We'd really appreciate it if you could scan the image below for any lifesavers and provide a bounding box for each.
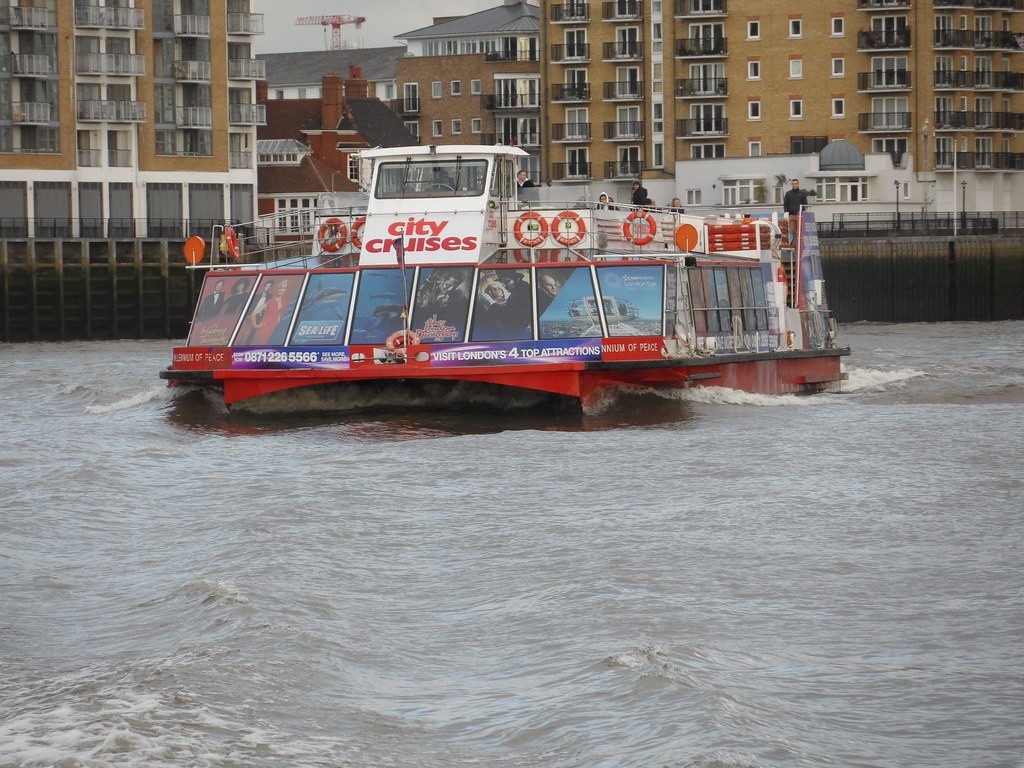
[514,250,548,263]
[226,227,240,259]
[386,330,420,354]
[350,217,366,249]
[317,218,347,253]
[551,249,584,262]
[623,212,656,245]
[514,212,548,247]
[551,211,585,245]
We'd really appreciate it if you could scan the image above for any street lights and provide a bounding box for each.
[894,180,901,212]
[961,180,967,211]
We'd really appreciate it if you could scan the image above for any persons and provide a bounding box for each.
[669,200,683,214]
[596,192,610,210]
[572,196,588,211]
[431,268,564,311]
[607,197,617,211]
[631,180,648,209]
[513,170,535,188]
[434,172,457,191]
[195,277,290,347]
[784,180,809,217]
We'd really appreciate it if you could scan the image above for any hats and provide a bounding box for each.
[631,178,642,189]
[493,269,524,281]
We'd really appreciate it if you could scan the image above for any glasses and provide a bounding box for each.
[500,279,512,284]
[279,287,287,289]
[442,176,448,178]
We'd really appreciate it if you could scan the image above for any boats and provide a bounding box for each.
[157,137,850,419]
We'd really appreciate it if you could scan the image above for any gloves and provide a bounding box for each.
[783,212,790,223]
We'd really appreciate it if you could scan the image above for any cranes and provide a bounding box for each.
[294,15,365,51]
